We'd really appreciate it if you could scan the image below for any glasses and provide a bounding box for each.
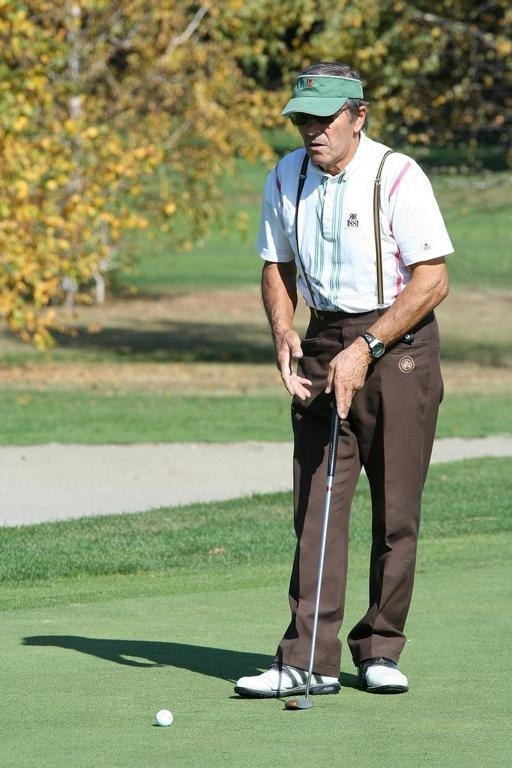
[286,106,358,128]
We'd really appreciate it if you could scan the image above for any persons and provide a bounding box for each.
[231,62,455,702]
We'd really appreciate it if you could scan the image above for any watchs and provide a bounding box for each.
[360,330,387,361]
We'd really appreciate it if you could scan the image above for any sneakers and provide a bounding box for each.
[232,659,344,699]
[358,655,411,694]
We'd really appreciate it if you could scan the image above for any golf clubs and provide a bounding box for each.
[285,389,340,710]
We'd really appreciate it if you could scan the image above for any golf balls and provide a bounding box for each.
[156,709,173,726]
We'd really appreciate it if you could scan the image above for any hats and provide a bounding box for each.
[281,72,365,119]
